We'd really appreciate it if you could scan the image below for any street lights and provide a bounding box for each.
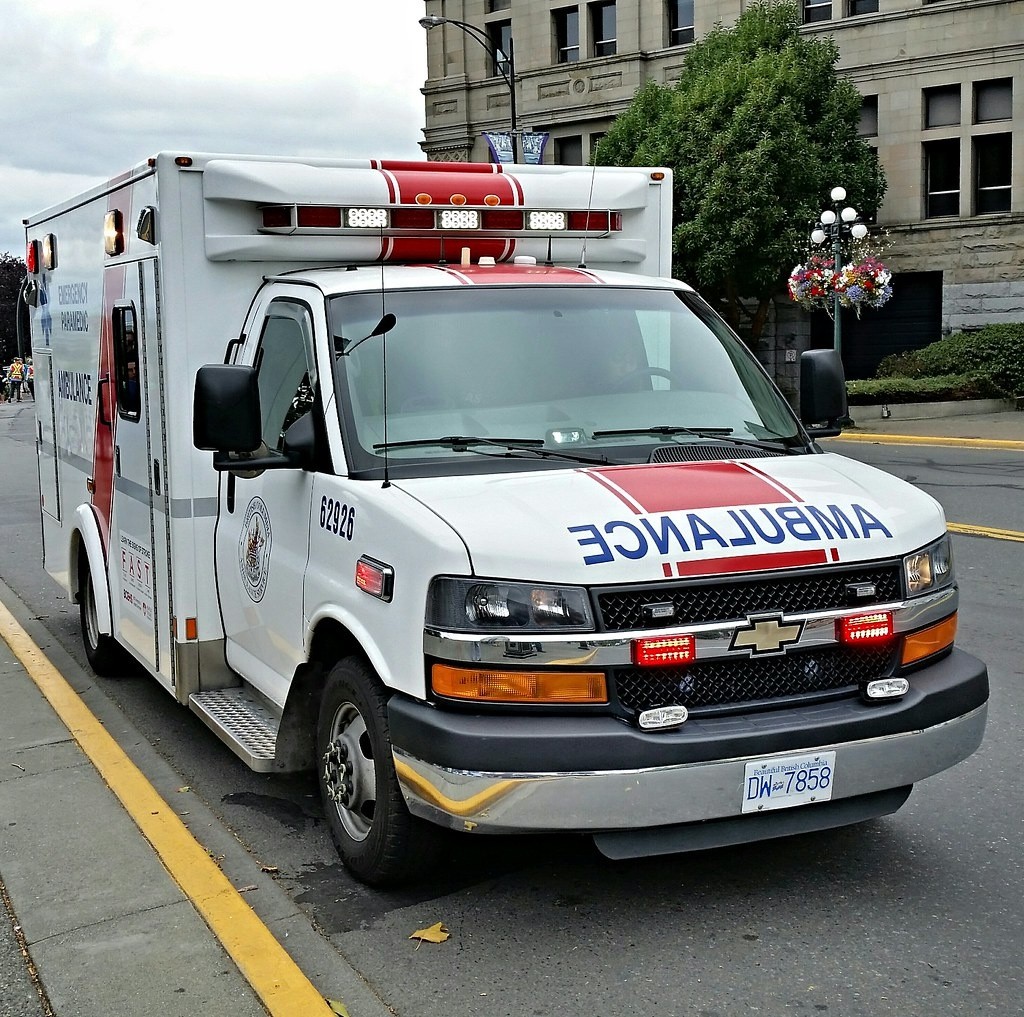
[418,17,517,164]
[811,187,867,359]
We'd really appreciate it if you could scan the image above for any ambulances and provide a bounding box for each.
[21,152,987,891]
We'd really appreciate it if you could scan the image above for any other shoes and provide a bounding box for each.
[17,400,22,402]
[22,391,29,394]
[8,397,11,403]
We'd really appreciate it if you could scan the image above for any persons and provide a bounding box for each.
[0,356,35,402]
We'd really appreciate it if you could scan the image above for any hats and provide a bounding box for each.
[14,357,20,361]
[28,359,33,364]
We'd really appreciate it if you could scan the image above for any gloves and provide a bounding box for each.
[21,377,24,381]
[8,377,11,380]
